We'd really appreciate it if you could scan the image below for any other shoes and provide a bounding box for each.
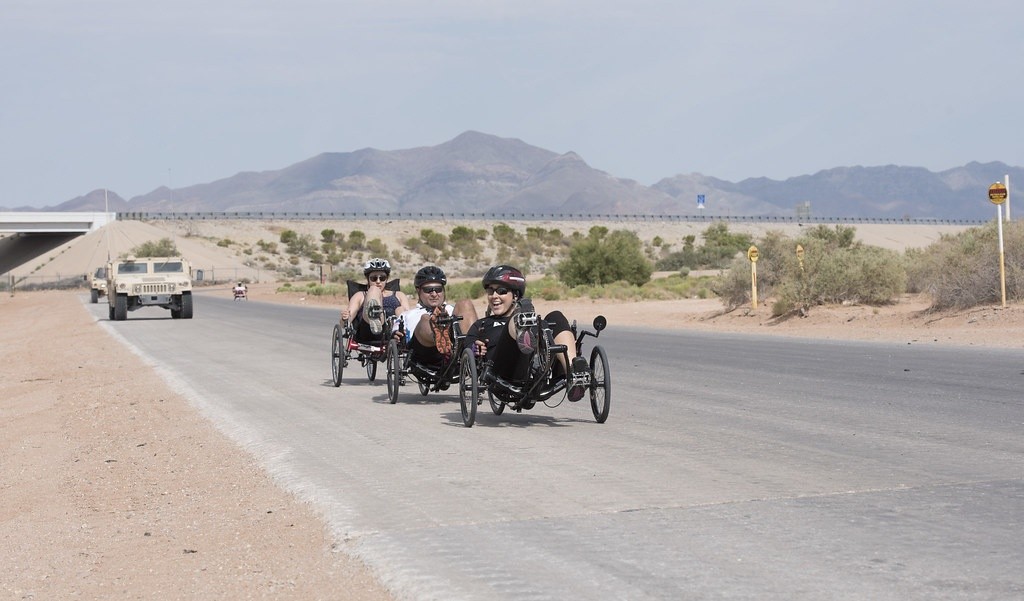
[366,298,383,335]
[429,305,451,354]
[567,357,589,402]
[514,298,538,355]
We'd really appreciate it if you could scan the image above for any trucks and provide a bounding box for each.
[104,254,194,320]
[89,266,108,304]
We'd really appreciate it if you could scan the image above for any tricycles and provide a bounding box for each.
[379,305,508,416]
[233,285,248,301]
[458,313,612,428]
[330,277,411,388]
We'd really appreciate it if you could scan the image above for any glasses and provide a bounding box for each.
[485,286,512,295]
[420,286,445,292]
[367,275,389,281]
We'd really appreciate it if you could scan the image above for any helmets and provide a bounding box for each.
[482,265,525,297]
[364,258,390,276]
[414,266,447,289]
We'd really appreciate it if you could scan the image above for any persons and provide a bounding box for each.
[234,282,245,290]
[339,258,410,350]
[391,266,480,367]
[463,265,590,402]
